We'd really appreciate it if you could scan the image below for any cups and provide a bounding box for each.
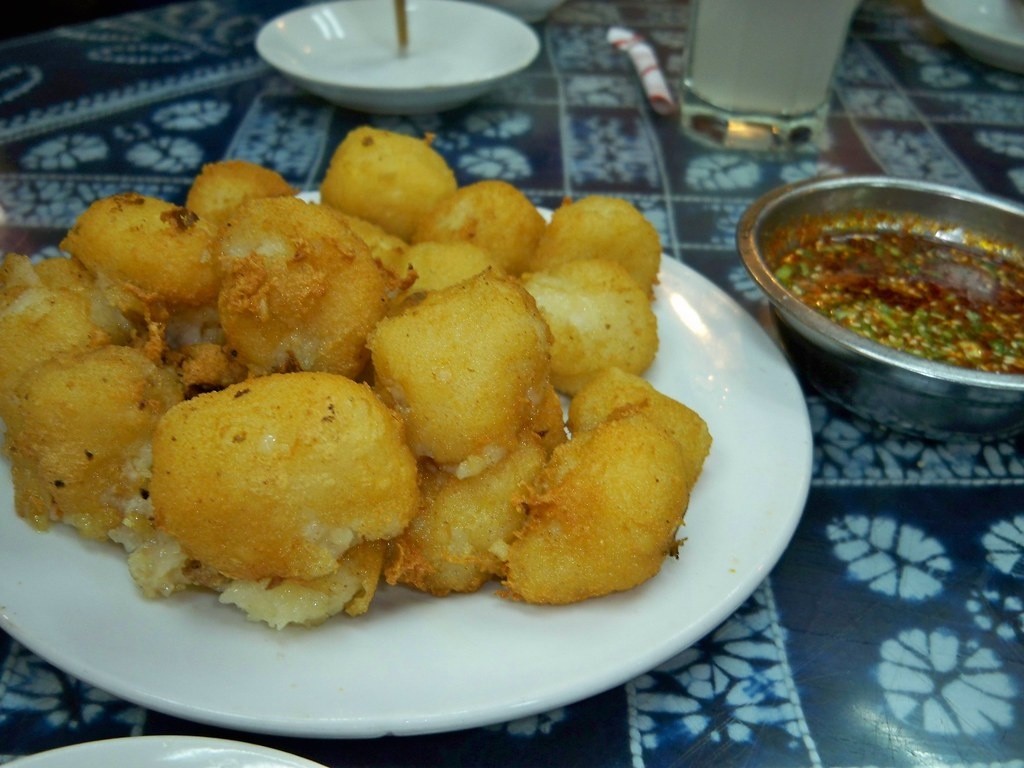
[679,0,861,153]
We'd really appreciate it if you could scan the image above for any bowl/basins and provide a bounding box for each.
[736,172,1024,442]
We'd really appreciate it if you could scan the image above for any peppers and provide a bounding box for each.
[773,237,1024,377]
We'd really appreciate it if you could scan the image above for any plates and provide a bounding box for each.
[0,197,816,741]
[921,0,1024,75]
[0,735,326,768]
[253,0,541,116]
